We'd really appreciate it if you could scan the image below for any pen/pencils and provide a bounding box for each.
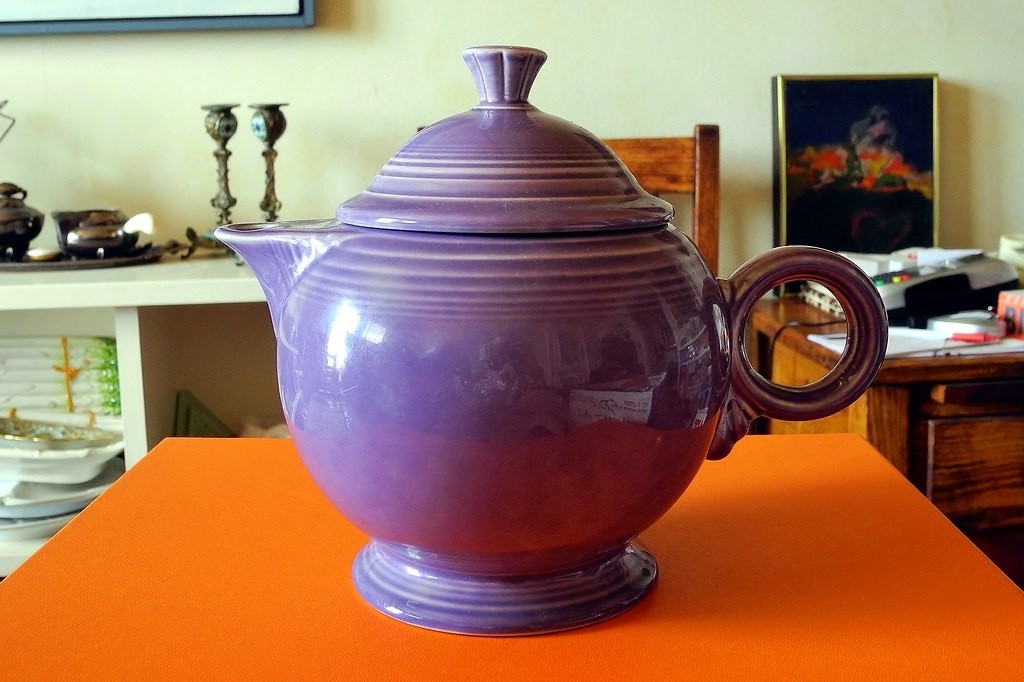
[887,328,998,342]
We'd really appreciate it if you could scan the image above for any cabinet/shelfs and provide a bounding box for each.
[0,251,270,582]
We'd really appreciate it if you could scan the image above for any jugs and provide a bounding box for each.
[213,44,888,636]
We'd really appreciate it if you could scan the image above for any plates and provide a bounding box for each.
[0,515,81,540]
[0,457,126,519]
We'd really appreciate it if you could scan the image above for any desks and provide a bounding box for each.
[744,297,1022,537]
[0,433,1024,682]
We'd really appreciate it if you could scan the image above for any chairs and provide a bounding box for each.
[416,125,720,279]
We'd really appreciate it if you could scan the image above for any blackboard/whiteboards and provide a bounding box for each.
[0,0,319,33]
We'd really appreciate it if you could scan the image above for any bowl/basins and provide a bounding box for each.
[0,409,124,484]
